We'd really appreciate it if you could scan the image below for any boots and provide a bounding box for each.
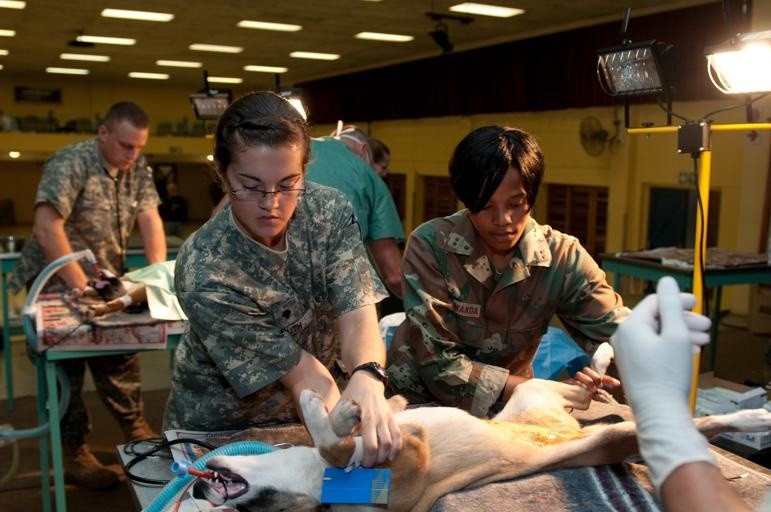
[59,436,121,488]
[125,419,160,439]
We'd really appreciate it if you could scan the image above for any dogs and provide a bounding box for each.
[66,269,148,319]
[191,340,771,512]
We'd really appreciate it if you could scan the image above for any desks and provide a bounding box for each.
[601,247,769,367]
[19,305,187,512]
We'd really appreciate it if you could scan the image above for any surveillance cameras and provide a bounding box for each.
[426,31,453,53]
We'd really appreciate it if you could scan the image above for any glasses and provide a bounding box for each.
[218,160,310,204]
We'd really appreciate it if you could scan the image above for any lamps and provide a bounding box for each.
[705,27,771,141]
[586,41,684,124]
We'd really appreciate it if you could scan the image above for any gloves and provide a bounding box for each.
[608,275,721,499]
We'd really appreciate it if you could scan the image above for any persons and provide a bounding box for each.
[19,100,166,488]
[391,124,647,420]
[604,261,749,511]
[161,88,399,464]
[369,139,393,176]
[305,123,410,301]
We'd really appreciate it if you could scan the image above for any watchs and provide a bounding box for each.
[352,359,389,384]
